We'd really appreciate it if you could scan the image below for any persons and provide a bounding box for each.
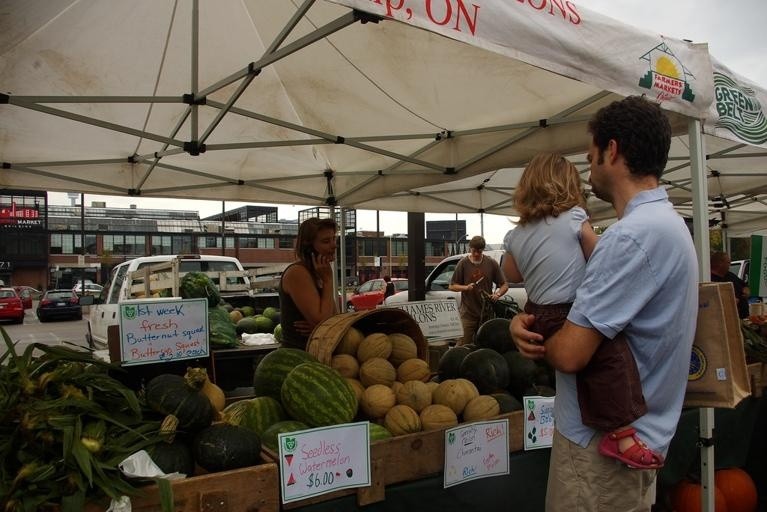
[499,152,665,469]
[383,275,395,298]
[709,251,750,320]
[508,94,699,512]
[280,217,339,347]
[447,236,510,346]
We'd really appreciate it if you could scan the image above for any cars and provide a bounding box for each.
[720,250,753,283]
[1,274,103,324]
[335,274,410,313]
[386,249,530,312]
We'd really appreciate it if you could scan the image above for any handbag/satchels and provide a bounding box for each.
[682,282,752,409]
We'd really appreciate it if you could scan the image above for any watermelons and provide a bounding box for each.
[209,308,237,347]
[264,306,277,321]
[331,324,501,437]
[241,305,254,317]
[181,272,220,307]
[236,317,257,336]
[218,298,233,311]
[223,348,392,455]
[257,315,274,331]
[274,324,284,343]
[437,317,555,413]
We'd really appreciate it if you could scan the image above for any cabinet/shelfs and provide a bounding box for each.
[40,324,767,512]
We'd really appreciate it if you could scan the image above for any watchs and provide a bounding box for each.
[496,292,500,297]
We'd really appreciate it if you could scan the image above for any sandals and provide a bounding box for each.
[598,427,665,469]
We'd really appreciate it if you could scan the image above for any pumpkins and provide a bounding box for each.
[714,464,758,512]
[126,367,260,486]
[670,479,727,512]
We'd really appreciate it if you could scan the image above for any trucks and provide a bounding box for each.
[86,253,257,354]
